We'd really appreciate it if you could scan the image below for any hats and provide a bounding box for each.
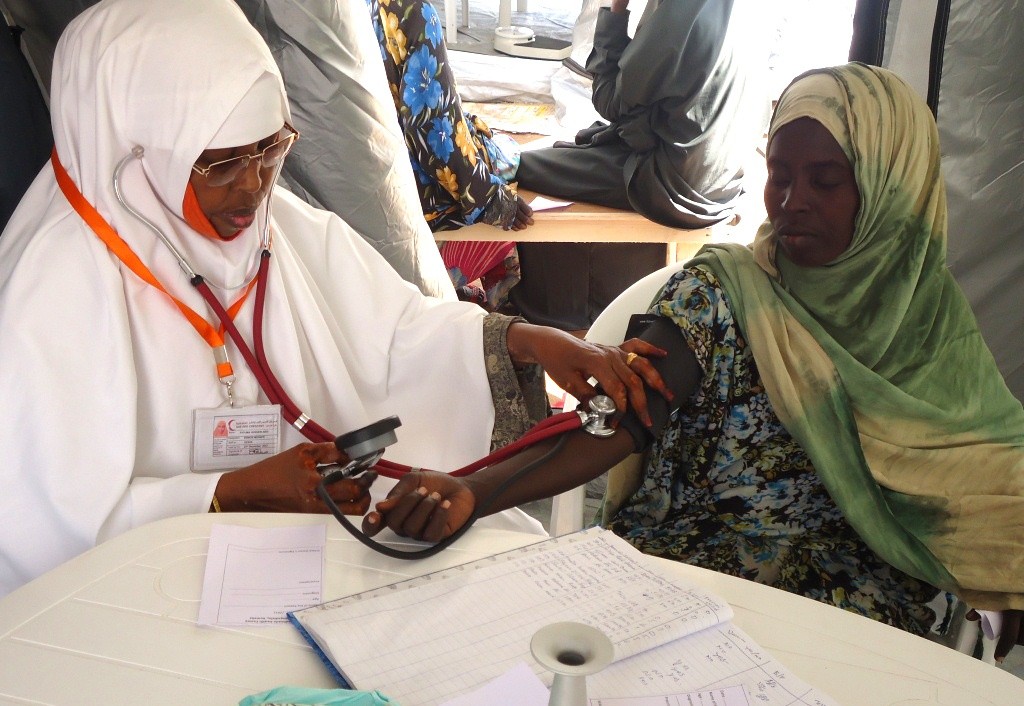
[200,72,285,150]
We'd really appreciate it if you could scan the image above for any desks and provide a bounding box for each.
[420,96,708,269]
[0,512,1024,706]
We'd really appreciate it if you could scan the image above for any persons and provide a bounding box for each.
[364,0,534,231]
[515,0,746,230]
[0,0,674,599]
[362,61,1024,662]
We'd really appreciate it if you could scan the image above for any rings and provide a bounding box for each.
[627,352,637,365]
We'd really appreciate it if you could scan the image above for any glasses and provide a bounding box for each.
[191,123,300,187]
